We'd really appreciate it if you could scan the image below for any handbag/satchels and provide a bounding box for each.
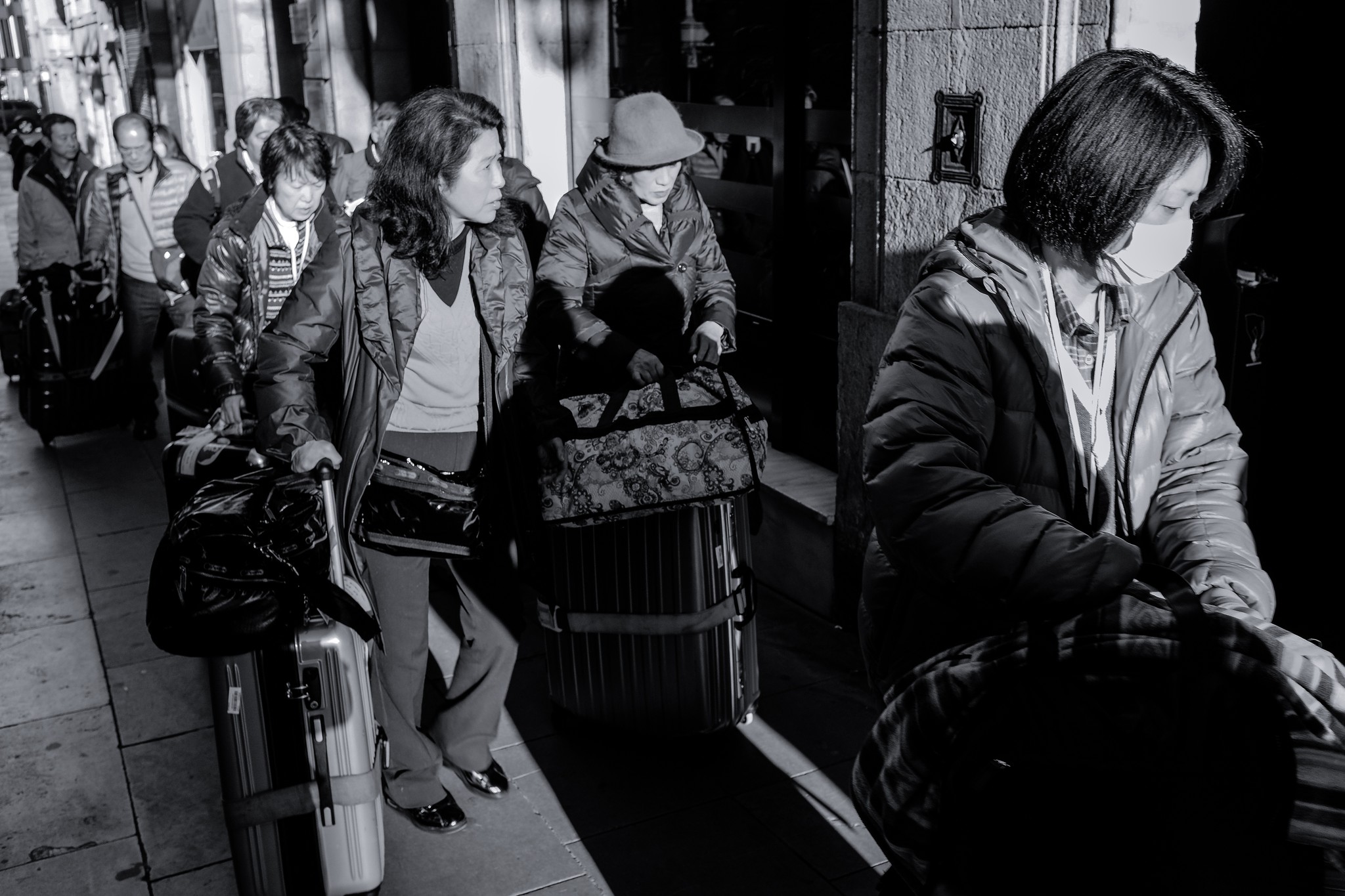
[146,465,331,660]
[526,355,770,532]
[847,563,1302,889]
[351,395,518,562]
[125,169,190,294]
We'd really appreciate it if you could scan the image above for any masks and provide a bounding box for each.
[1094,219,1194,288]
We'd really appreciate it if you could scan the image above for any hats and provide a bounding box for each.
[594,92,706,166]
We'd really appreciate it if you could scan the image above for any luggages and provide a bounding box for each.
[160,406,269,523]
[513,495,761,737]
[211,467,390,896]
[0,258,161,449]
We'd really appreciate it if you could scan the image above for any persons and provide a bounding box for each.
[10,94,554,427]
[531,89,741,390]
[850,47,1277,722]
[239,88,536,837]
